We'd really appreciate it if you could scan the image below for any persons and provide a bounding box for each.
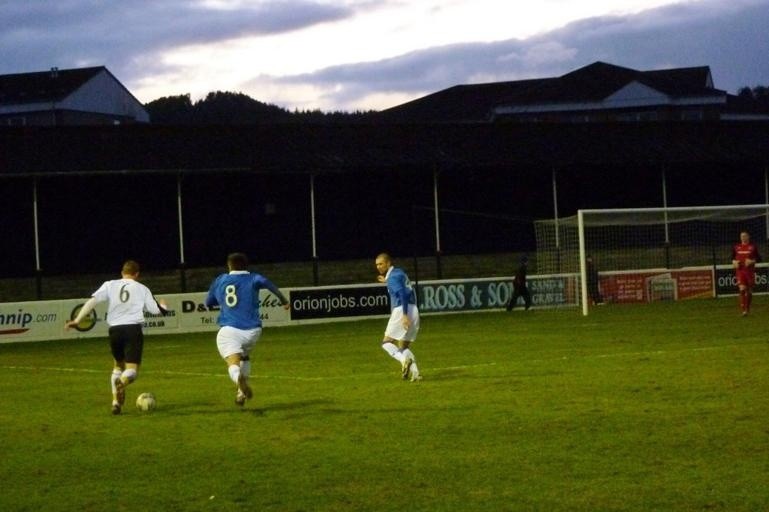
[506,256,535,312]
[731,231,762,317]
[64,261,167,415]
[205,253,290,406]
[373,252,424,382]
[586,254,605,306]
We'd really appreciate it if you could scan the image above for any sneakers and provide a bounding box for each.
[236,373,252,405]
[401,357,423,382]
[111,377,125,414]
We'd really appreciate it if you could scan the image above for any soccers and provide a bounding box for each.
[135,393,156,414]
